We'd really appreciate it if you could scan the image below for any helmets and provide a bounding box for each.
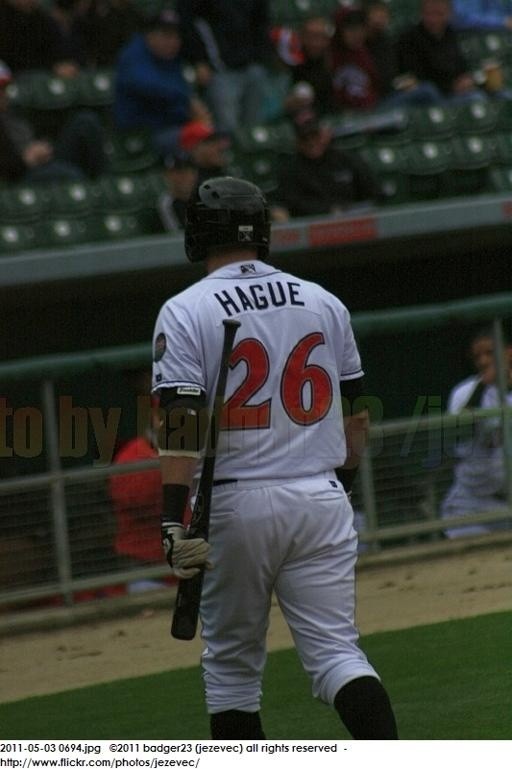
[183,175,273,264]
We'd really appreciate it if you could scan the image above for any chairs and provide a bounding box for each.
[0,0,512,257]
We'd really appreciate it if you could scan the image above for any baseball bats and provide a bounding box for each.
[170,320,243,640]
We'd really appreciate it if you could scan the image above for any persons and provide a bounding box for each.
[1,0,509,236]
[106,388,176,592]
[150,171,399,740]
[438,329,512,543]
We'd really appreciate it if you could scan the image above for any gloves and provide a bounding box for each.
[160,520,216,579]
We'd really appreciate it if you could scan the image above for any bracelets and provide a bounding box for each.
[159,480,191,525]
